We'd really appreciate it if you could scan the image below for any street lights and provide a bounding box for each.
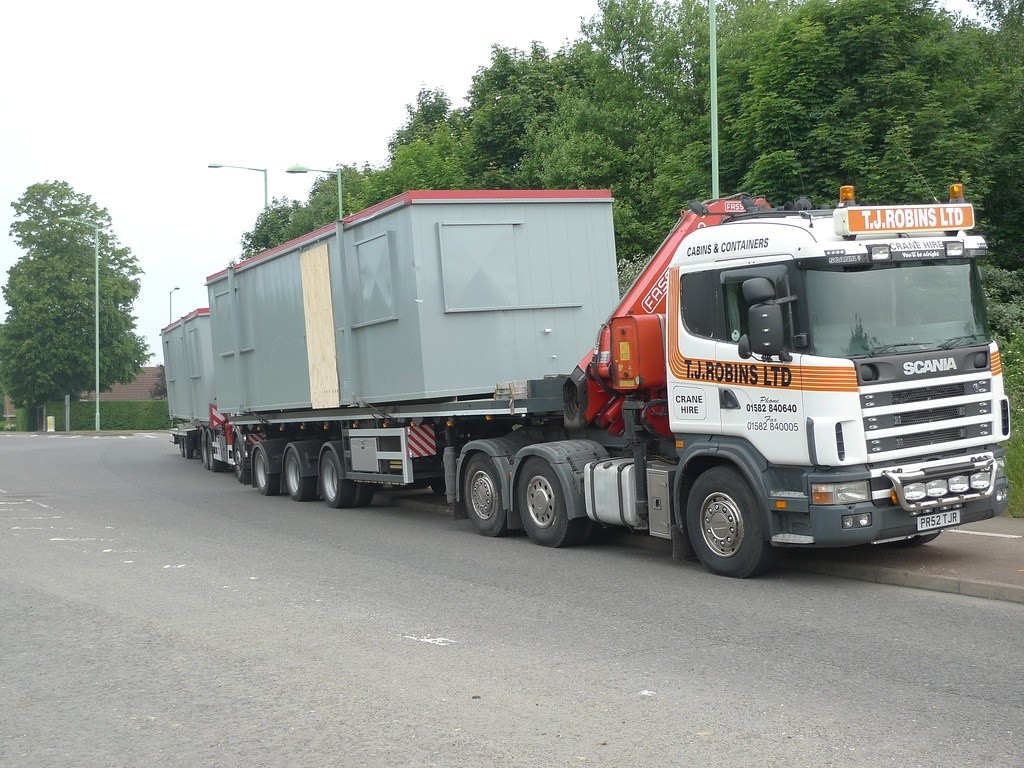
[210,163,268,215]
[285,167,344,218]
[170,287,178,323]
[58,218,100,434]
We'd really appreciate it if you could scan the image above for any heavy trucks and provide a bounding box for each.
[160,188,1011,582]
